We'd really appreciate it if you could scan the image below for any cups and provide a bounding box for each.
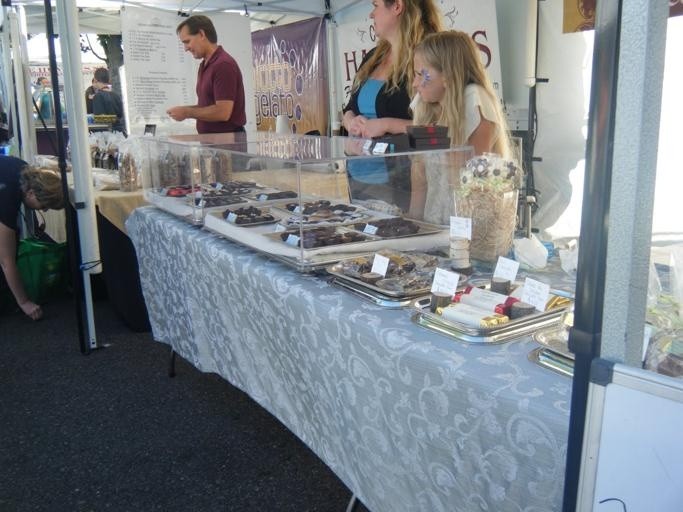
[276,115,289,135]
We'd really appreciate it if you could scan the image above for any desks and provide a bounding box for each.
[124,204,575,510]
[33,160,350,237]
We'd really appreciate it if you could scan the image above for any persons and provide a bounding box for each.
[31,76,56,121]
[340,0,444,217]
[165,15,248,182]
[406,29,513,228]
[0,154,64,321]
[92,68,127,137]
[85,78,95,114]
